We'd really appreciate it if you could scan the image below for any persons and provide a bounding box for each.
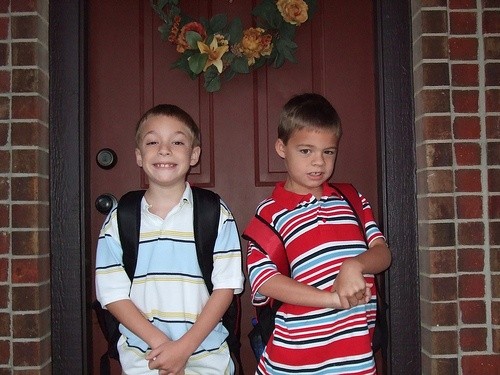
[96,103,245,375]
[239,91,393,375]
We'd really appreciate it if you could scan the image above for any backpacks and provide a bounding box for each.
[90,184,242,375]
[241,181,370,368]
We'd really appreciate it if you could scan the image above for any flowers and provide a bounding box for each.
[159,1,316,91]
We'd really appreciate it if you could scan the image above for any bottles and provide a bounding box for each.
[251,318,264,362]
